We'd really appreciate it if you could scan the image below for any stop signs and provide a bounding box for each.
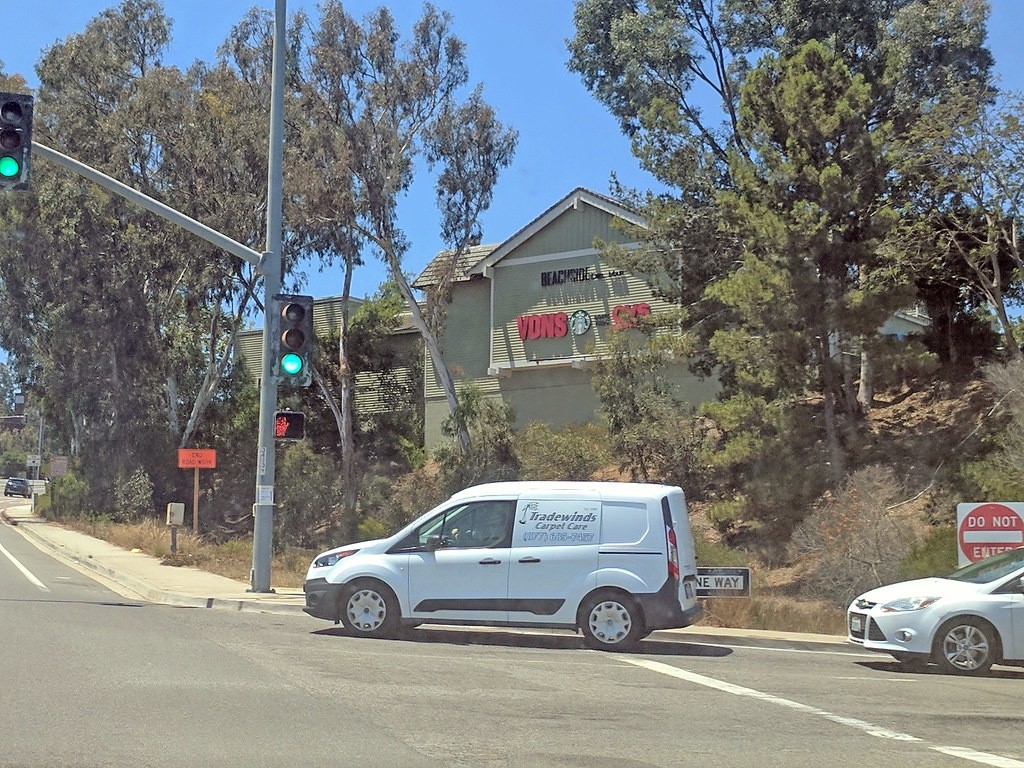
[956,502,1024,569]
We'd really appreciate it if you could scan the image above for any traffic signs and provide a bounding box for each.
[695,565,751,599]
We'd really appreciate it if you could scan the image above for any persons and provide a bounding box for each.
[452,512,507,546]
[45,477,51,486]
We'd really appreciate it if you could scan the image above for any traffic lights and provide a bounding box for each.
[276,292,313,388]
[0,93,35,190]
[272,411,306,444]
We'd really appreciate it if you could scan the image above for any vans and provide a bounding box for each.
[302,481,705,653]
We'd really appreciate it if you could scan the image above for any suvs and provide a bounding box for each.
[4,478,33,498]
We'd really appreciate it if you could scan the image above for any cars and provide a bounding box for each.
[846,547,1024,677]
